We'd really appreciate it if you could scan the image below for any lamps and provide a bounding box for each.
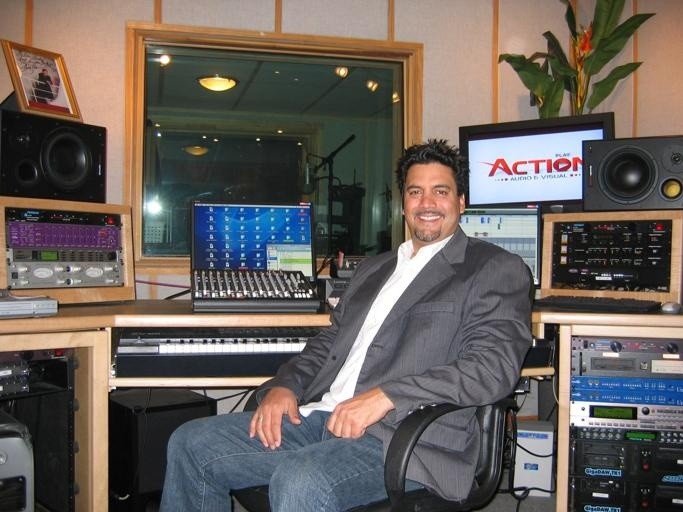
[184,145,208,156]
[195,75,238,92]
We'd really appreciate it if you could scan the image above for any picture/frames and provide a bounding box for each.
[0,39,83,123]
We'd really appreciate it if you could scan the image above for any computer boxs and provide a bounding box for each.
[0,409,37,512]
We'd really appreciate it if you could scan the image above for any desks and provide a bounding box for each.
[0,298,682,512]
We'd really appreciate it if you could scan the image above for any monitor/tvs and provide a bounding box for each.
[459,111,615,214]
[458,208,541,294]
[189,198,317,287]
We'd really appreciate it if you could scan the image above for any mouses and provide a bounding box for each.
[663,300,682,314]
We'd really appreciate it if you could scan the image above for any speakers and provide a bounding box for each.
[1,107,108,204]
[581,134,683,212]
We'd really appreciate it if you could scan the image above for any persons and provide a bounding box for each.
[160,138,534,511]
[35,68,54,103]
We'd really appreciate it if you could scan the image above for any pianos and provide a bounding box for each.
[115,326,321,378]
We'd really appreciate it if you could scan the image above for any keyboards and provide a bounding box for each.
[532,294,661,314]
